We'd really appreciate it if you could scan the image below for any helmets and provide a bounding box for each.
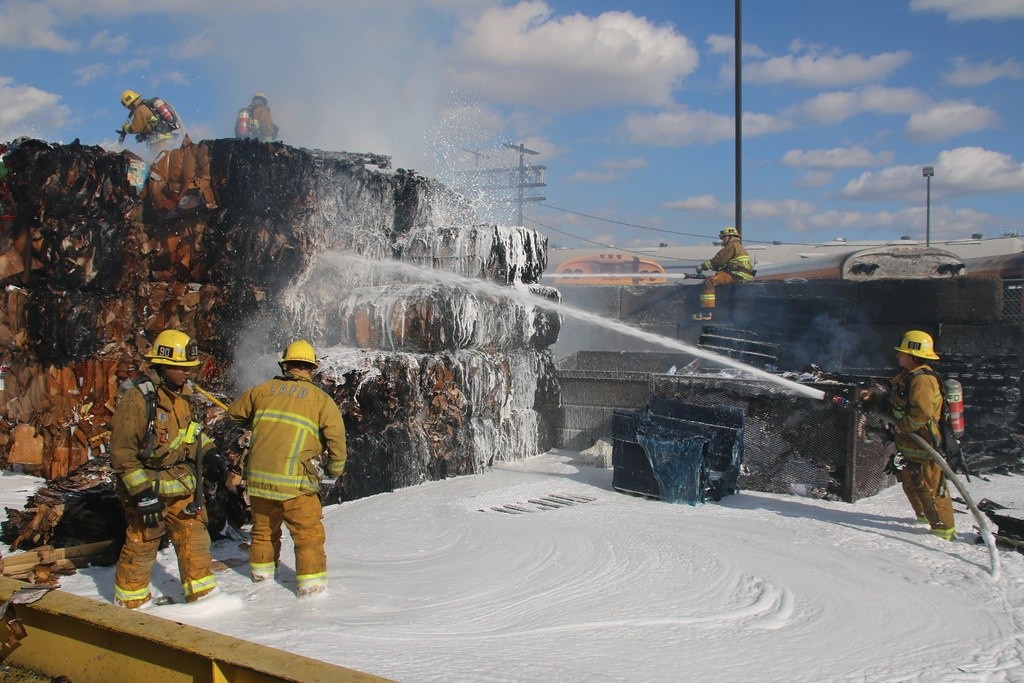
[894,329,941,360]
[719,227,741,240]
[120,91,141,110]
[252,92,268,106]
[278,339,319,369]
[145,329,200,367]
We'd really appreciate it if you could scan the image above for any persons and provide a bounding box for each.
[118,90,178,159]
[108,329,220,612]
[235,93,277,143]
[225,339,347,595]
[691,226,757,321]
[860,329,961,542]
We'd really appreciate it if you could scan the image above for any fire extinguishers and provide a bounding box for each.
[944,378,966,444]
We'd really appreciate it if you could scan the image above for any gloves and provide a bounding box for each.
[320,475,335,500]
[137,492,165,529]
[206,447,229,476]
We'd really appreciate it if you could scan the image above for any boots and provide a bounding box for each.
[691,308,713,321]
